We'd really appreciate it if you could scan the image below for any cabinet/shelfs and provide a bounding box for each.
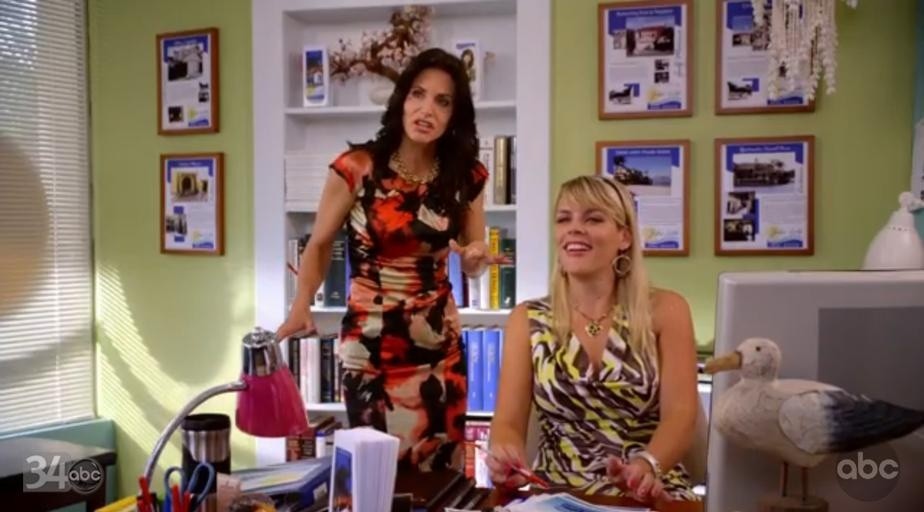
[283,97,520,425]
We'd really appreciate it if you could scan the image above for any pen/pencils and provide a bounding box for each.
[135,477,191,512]
[476,443,550,489]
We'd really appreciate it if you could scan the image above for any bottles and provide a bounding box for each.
[863,192,924,272]
[181,414,231,494]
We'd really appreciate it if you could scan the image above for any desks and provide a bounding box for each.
[0,435,119,512]
[477,484,707,511]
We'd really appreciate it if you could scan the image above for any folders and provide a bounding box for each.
[231,459,329,508]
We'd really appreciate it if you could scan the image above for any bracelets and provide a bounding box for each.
[631,450,665,481]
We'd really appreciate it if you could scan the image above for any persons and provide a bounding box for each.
[461,49,473,78]
[484,177,708,510]
[274,48,511,472]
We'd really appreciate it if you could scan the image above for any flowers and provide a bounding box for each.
[324,21,433,90]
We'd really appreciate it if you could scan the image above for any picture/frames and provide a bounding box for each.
[711,134,816,260]
[157,151,226,257]
[596,1,697,122]
[153,25,220,137]
[712,0,820,115]
[591,136,694,259]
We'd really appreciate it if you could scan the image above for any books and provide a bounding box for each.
[227,457,334,511]
[479,134,512,205]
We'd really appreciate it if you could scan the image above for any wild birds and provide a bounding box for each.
[704,337,924,506]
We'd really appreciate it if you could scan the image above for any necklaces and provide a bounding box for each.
[571,308,614,339]
[392,150,441,183]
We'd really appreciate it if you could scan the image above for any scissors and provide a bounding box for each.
[164,463,216,512]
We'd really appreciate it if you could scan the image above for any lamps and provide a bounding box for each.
[855,187,923,274]
[123,327,315,512]
